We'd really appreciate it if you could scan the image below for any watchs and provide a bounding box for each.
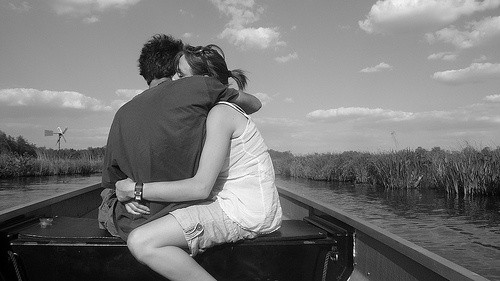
[135,181,143,202]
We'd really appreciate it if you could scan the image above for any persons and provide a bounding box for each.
[115,44,283,281]
[98,34,262,243]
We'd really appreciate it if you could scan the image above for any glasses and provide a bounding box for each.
[193,44,212,77]
